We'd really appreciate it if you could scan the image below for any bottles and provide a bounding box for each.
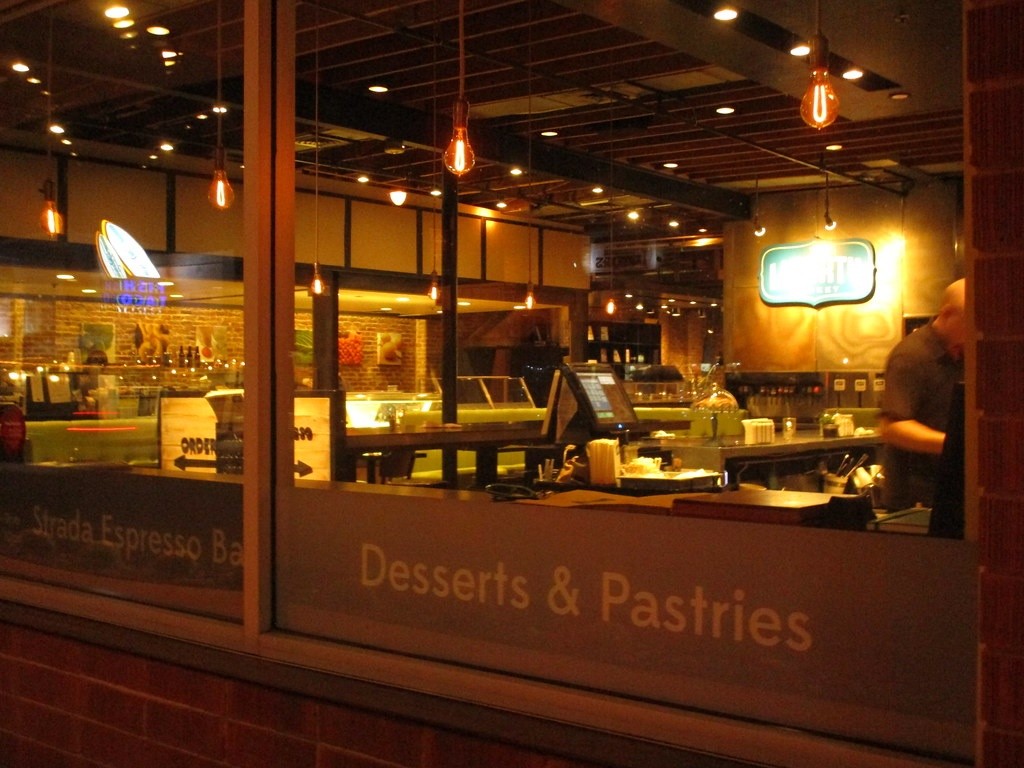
[179,345,200,369]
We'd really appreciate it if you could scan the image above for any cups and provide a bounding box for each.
[823,475,848,494]
[823,424,837,437]
[625,382,681,403]
[783,418,797,441]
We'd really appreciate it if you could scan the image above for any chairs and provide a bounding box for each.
[818,407,881,429]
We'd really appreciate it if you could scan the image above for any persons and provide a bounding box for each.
[80,351,115,418]
[881,276,964,511]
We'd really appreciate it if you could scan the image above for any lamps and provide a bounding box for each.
[443,0,475,180]
[310,0,325,296]
[800,0,841,135]
[207,0,236,213]
[429,44,441,301]
[824,172,837,231]
[606,25,616,318]
[752,177,766,237]
[38,0,66,241]
[523,1,537,314]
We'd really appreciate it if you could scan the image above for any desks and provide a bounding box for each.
[635,427,883,493]
[342,419,543,484]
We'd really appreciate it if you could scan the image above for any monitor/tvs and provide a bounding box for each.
[558,363,640,432]
